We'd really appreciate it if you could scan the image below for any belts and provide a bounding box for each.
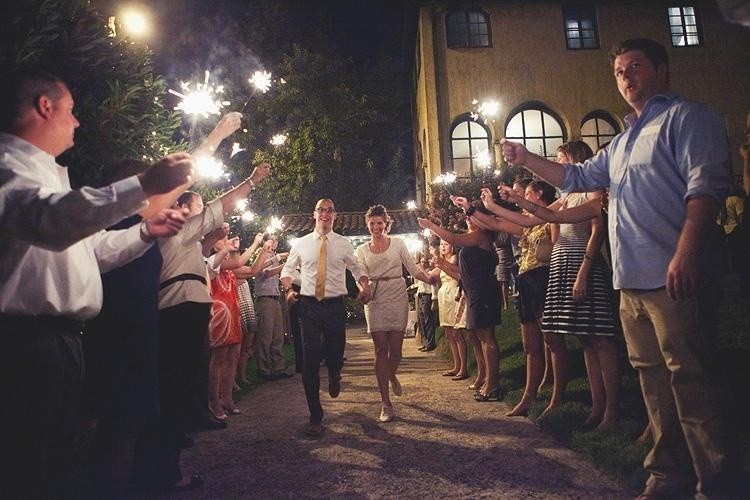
[300,293,347,305]
[417,292,432,296]
[0,314,88,340]
[257,295,281,301]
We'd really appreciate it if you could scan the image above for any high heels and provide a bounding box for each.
[467,378,486,390]
[505,400,533,418]
[472,386,505,402]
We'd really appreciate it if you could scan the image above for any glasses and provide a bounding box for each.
[315,206,335,214]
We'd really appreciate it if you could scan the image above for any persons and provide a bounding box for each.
[353,204,439,424]
[1,66,289,500]
[279,198,372,436]
[417,39,749,499]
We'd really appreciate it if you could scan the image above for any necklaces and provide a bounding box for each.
[371,238,388,250]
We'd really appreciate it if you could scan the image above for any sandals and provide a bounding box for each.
[451,373,468,381]
[158,472,207,494]
[390,376,404,397]
[441,368,457,376]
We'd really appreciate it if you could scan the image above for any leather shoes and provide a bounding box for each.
[208,402,231,421]
[221,401,243,414]
[275,370,296,379]
[258,373,280,381]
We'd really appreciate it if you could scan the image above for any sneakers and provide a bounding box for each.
[191,413,228,431]
[417,345,437,353]
[379,402,395,423]
[305,417,323,436]
[329,369,341,398]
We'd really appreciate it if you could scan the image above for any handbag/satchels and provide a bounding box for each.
[534,237,555,264]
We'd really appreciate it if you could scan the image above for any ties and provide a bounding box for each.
[315,235,328,302]
